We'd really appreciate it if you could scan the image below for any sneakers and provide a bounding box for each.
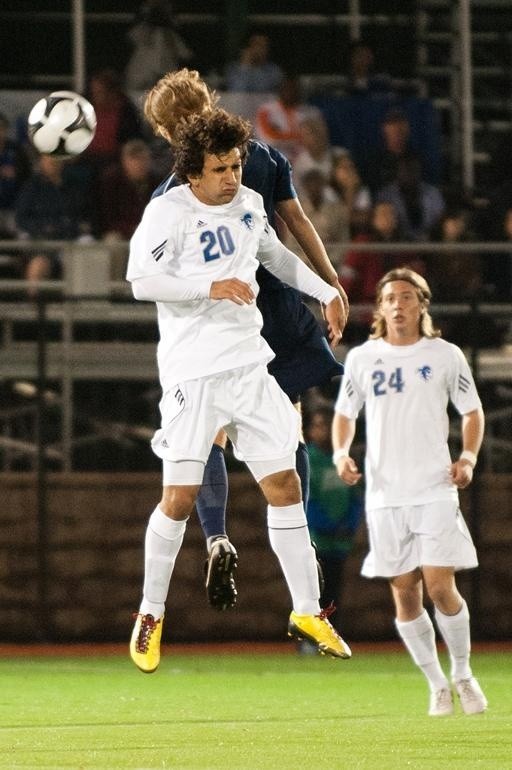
[128,606,166,675]
[426,685,455,718]
[450,675,488,716]
[310,540,327,596]
[203,535,240,613]
[286,608,352,662]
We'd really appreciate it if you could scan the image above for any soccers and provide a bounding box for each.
[26,91,98,159]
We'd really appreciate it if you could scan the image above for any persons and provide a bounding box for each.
[139,65,351,617]
[117,111,357,673]
[291,401,364,660]
[0,4,507,475]
[326,265,492,719]
